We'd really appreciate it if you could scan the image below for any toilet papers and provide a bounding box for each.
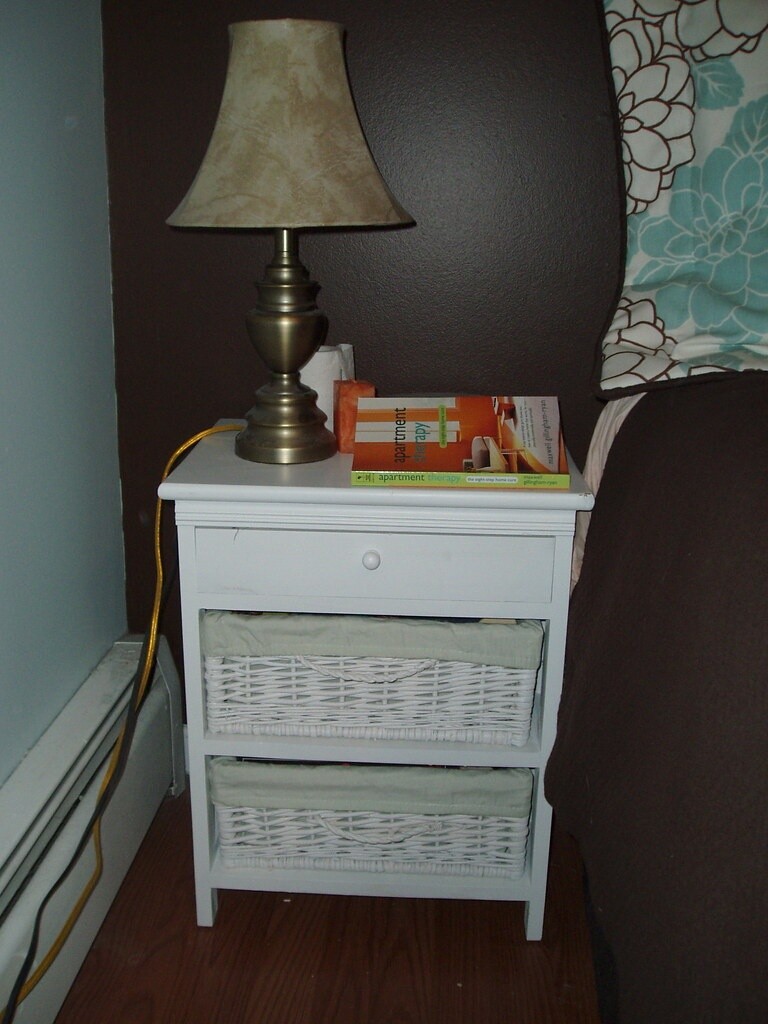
[301,342,355,434]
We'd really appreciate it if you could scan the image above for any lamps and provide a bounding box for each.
[163,16,417,466]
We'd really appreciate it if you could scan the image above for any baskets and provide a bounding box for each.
[203,610,542,748]
[211,761,534,878]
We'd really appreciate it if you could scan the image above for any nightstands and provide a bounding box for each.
[157,419,595,942]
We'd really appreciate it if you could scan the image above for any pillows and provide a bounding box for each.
[593,0,768,404]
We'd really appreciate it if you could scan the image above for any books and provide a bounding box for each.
[351,396,571,489]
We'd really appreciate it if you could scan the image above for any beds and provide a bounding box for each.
[544,379,768,1024]
